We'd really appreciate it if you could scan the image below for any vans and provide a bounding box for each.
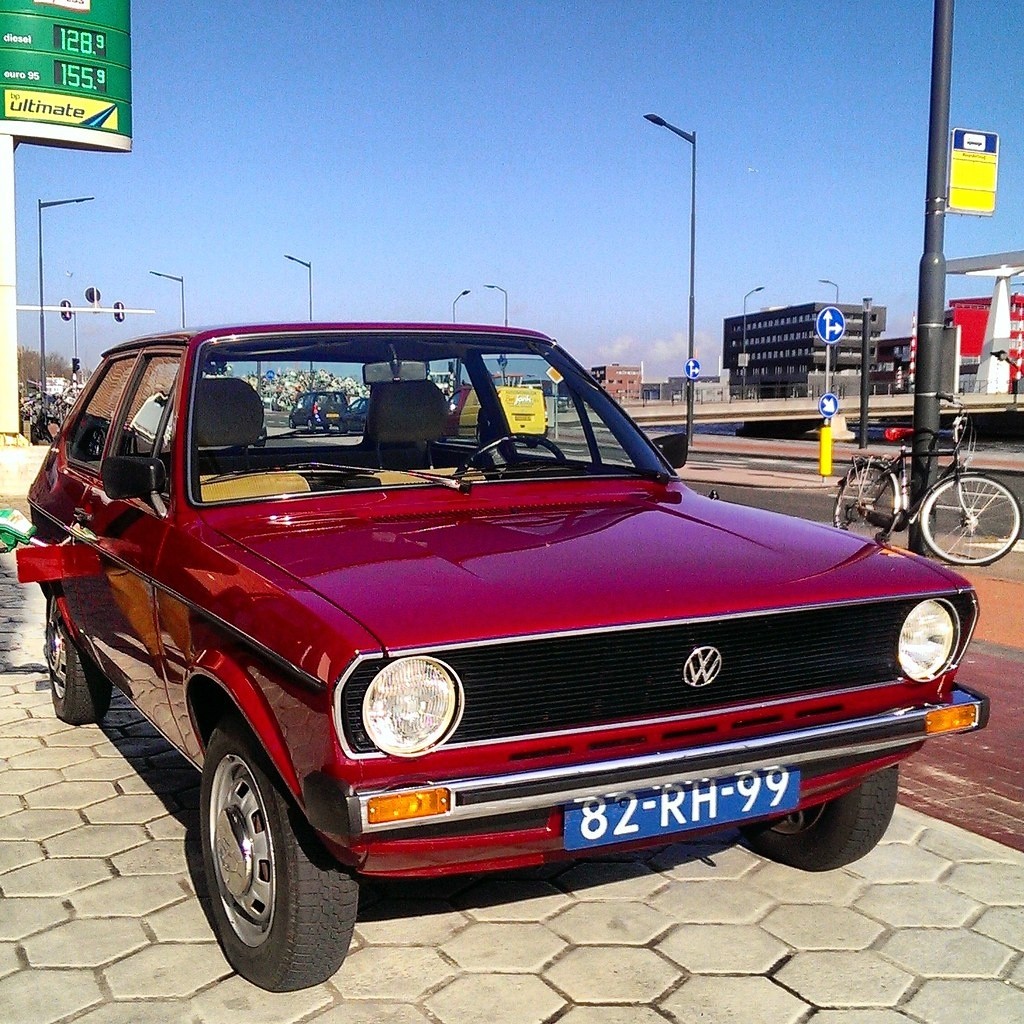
[288,391,349,433]
[441,386,548,447]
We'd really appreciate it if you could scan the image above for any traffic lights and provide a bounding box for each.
[72,358,80,373]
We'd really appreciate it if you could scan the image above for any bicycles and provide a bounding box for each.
[833,392,1023,566]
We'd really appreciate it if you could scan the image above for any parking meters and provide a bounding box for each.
[819,278,839,395]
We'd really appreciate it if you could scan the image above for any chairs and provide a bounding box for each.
[356,380,487,484]
[196,378,311,502]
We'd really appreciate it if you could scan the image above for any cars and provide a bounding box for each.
[17,320,992,993]
[339,397,370,434]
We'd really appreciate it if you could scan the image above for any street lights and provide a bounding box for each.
[284,254,312,391]
[741,286,764,399]
[484,283,507,385]
[453,289,471,386]
[150,270,185,329]
[643,113,696,445]
[39,196,96,405]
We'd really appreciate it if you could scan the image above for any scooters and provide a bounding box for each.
[29,406,54,445]
